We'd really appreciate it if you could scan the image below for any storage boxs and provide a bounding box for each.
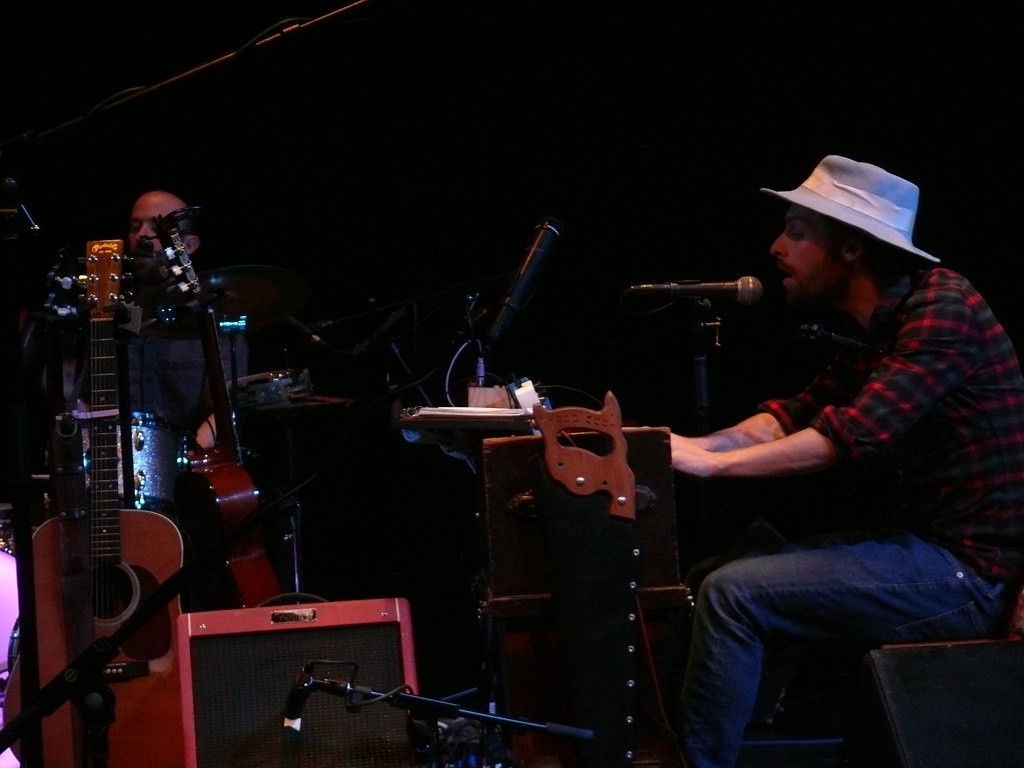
[475,427,697,768]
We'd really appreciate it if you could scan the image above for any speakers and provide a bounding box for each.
[479,426,681,591]
[486,585,694,768]
[177,595,419,768]
[844,636,1024,768]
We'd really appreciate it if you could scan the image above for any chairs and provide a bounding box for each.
[869,582,1024,768]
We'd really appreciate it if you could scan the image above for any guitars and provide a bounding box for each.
[142,202,282,606]
[2,236,197,767]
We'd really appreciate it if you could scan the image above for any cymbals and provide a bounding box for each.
[145,261,311,340]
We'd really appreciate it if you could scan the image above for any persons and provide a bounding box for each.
[41,191,248,613]
[670,151,1024,768]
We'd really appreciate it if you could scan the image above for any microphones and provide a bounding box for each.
[285,659,314,720]
[633,276,763,305]
[352,305,412,356]
[483,219,565,351]
[0,177,17,192]
[287,317,327,349]
[18,200,41,233]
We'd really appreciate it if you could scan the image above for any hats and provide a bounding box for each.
[761,154,938,264]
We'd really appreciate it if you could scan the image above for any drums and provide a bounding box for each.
[24,306,253,419]
[46,408,191,524]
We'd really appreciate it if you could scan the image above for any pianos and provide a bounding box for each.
[400,390,689,758]
